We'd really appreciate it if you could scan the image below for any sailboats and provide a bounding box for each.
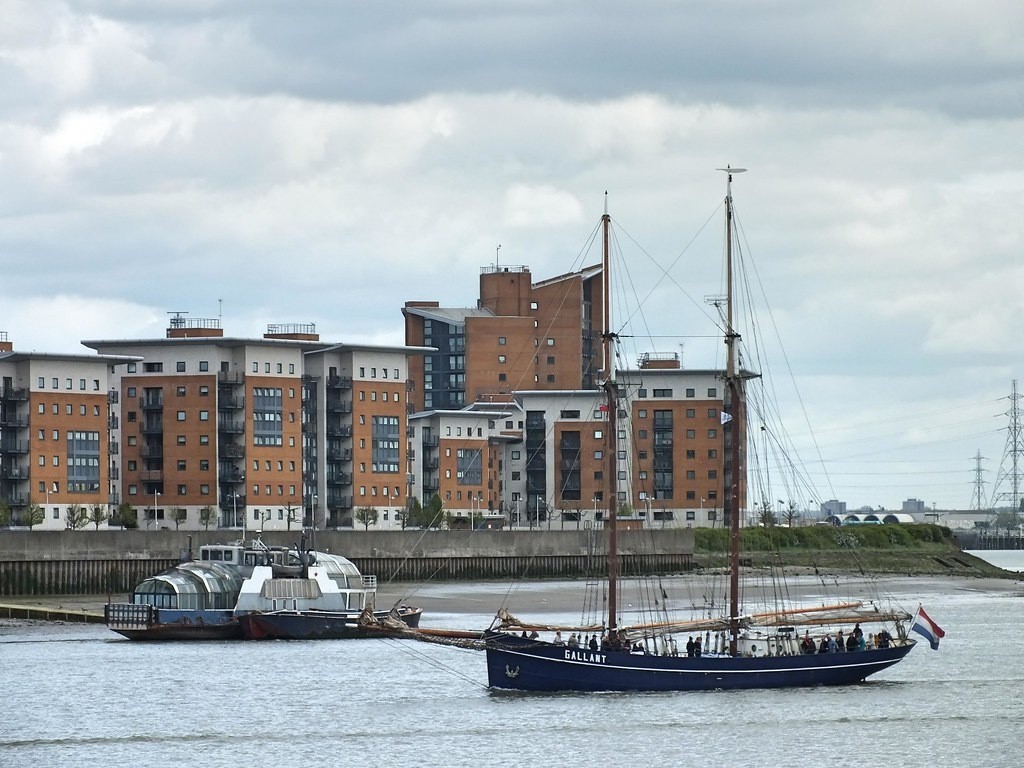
[488,171,918,697]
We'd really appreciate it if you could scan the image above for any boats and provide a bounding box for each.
[239,607,424,640]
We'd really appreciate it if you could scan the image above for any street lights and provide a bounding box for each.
[700,496,707,526]
[388,492,395,528]
[516,496,523,527]
[154,489,161,529]
[648,496,656,529]
[311,492,317,529]
[45,488,54,529]
[471,494,477,530]
[536,494,543,528]
[594,497,600,525]
[233,491,240,527]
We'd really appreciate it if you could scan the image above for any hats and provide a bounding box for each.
[571,633,576,637]
[592,634,597,637]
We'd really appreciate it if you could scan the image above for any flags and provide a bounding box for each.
[912,606,945,651]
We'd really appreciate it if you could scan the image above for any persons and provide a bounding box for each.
[685,635,704,657]
[554,632,645,656]
[802,623,895,655]
